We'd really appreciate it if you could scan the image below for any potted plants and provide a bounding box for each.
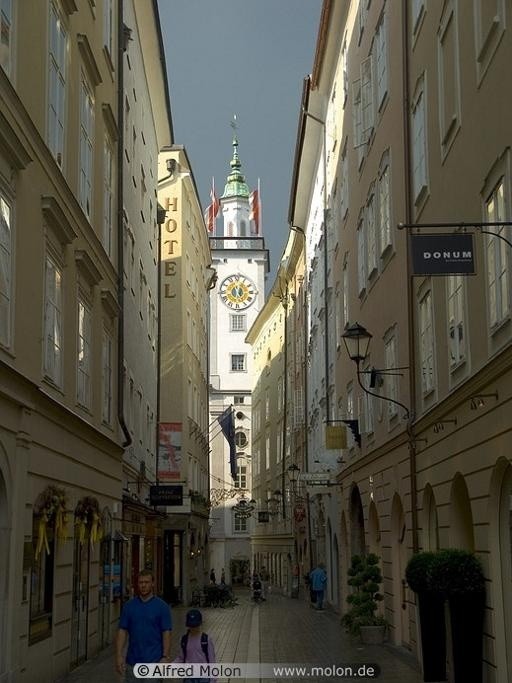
[340,551,390,644]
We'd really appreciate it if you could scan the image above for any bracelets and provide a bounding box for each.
[161,654,169,659]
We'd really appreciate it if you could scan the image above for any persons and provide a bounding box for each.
[209,564,270,601]
[218,405,236,480]
[115,568,171,682]
[308,560,328,608]
[170,609,219,682]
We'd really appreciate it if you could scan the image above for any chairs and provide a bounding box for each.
[193,583,238,608]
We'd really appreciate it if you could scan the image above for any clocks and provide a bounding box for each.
[216,271,261,311]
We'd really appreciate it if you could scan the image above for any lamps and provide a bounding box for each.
[339,320,411,422]
[287,461,315,505]
[466,388,499,411]
[430,414,457,435]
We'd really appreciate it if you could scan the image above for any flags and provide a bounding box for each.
[246,188,259,222]
[204,183,221,234]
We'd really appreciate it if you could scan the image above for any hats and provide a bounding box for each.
[186,609,202,626]
